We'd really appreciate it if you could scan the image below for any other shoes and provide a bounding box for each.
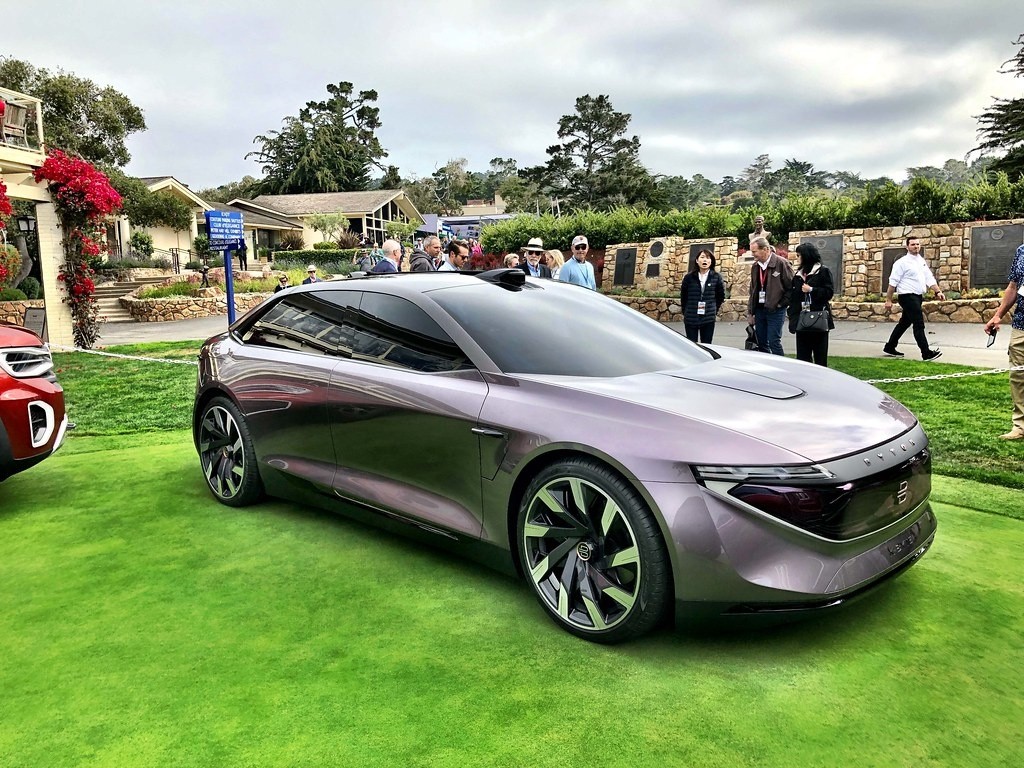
[998,431,1024,440]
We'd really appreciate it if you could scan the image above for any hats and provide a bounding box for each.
[572,236,588,246]
[374,243,378,246]
[360,250,366,254]
[521,237,546,251]
[307,265,316,271]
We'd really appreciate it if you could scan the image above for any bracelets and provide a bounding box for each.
[936,291,940,295]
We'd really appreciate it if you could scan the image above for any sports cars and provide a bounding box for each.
[190,266,937,646]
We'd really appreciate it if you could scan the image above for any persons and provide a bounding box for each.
[680,249,725,344]
[275,275,292,293]
[559,235,596,290]
[882,237,945,361]
[504,254,520,268]
[354,236,383,271]
[409,236,441,271]
[303,265,323,284]
[515,238,552,278]
[787,243,835,367]
[442,236,477,254]
[414,238,424,248]
[749,216,772,240]
[438,240,468,271]
[545,250,565,279]
[746,237,795,356]
[237,244,248,271]
[371,237,405,272]
[984,244,1024,439]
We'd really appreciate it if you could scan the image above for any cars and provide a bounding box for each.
[0,319,77,483]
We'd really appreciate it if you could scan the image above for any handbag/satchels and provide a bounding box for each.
[796,311,828,331]
[745,325,759,351]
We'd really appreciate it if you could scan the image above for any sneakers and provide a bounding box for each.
[882,343,904,356]
[922,350,942,361]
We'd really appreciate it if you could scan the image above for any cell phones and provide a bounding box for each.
[986,329,997,347]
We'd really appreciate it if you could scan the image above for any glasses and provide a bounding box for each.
[310,271,315,273]
[459,255,468,260]
[281,281,286,283]
[527,251,540,256]
[575,245,586,250]
